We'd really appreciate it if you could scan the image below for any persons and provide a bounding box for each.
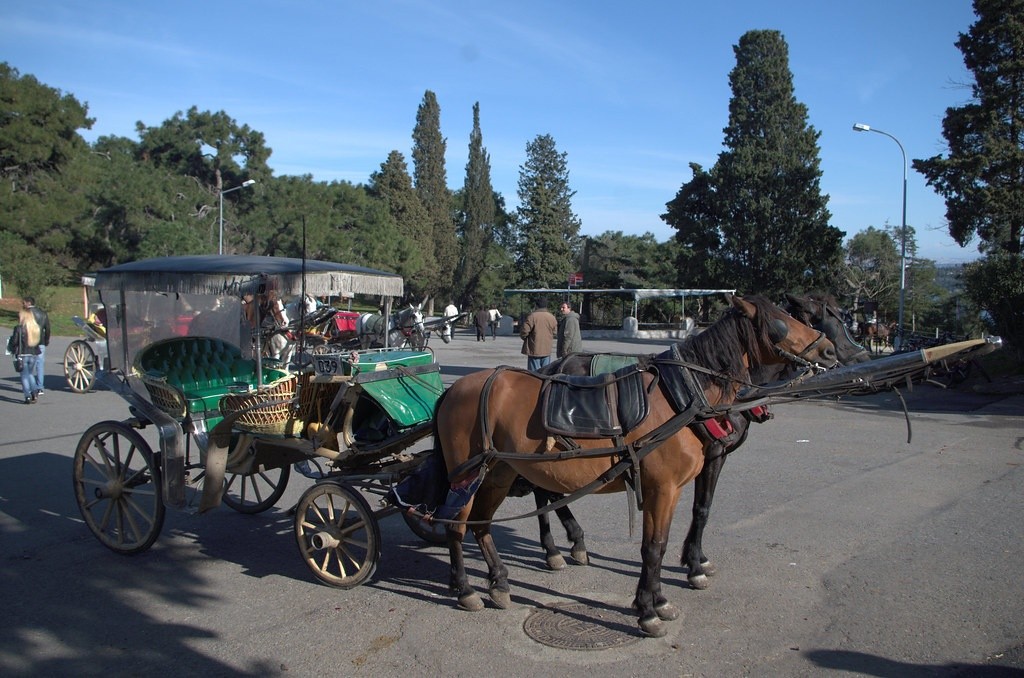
[22,297,50,394]
[489,303,501,341]
[483,307,491,338]
[557,301,583,359]
[474,305,487,341]
[444,300,459,339]
[674,311,682,340]
[6,307,42,404]
[520,296,557,371]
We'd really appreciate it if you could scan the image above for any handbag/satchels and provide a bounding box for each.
[496,319,500,328]
[13,357,23,372]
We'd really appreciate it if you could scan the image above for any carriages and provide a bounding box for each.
[64,250,1008,643]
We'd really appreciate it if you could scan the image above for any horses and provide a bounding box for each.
[242,290,289,347]
[433,295,840,636]
[286,294,319,320]
[530,294,873,591]
[864,321,897,354]
[354,295,430,355]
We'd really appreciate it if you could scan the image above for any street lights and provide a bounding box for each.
[218,180,257,257]
[853,122,908,338]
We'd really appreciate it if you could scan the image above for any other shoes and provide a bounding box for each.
[37,389,45,395]
[31,391,37,401]
[25,397,32,404]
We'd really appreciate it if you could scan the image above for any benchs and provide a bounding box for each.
[331,312,360,319]
[134,335,288,412]
[87,307,148,340]
[322,345,437,374]
